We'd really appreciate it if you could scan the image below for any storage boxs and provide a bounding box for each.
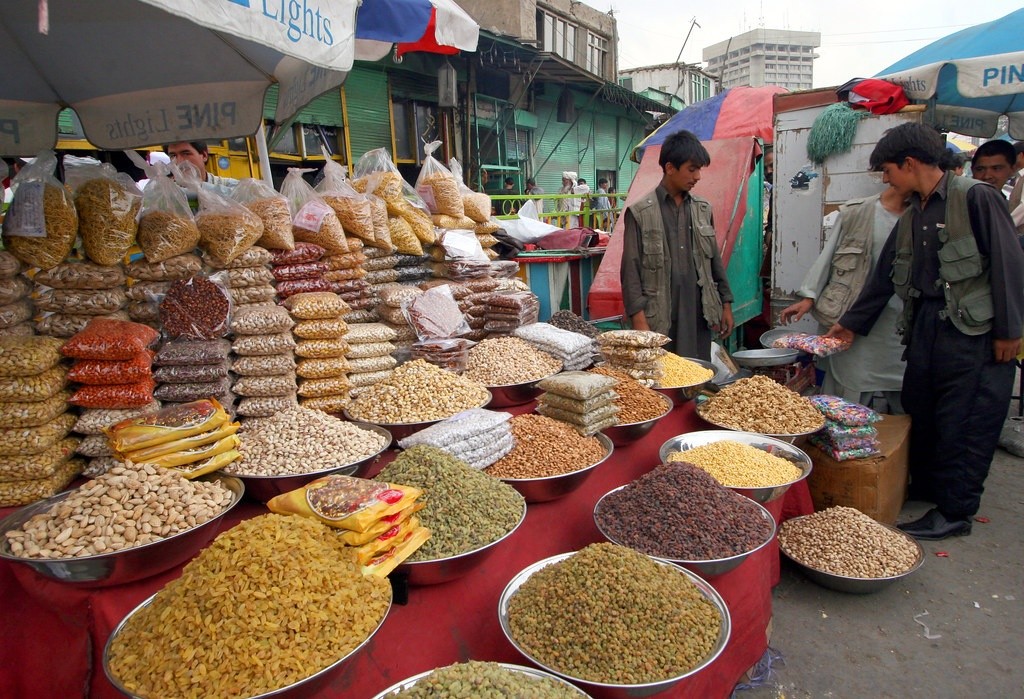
[791,412,911,525]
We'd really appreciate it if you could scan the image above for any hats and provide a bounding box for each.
[526,178,535,185]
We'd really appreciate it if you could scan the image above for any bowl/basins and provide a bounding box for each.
[217,420,393,508]
[483,366,563,409]
[694,399,827,447]
[649,357,720,406]
[343,391,492,452]
[496,551,732,699]
[600,390,674,448]
[776,515,926,595]
[659,430,813,504]
[370,662,594,699]
[101,593,392,699]
[394,493,527,585]
[496,432,614,503]
[593,483,776,581]
[0,475,245,589]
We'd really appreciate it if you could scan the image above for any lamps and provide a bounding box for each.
[439,55,459,109]
[557,83,574,124]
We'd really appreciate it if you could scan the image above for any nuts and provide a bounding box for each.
[108,512,393,699]
[506,541,721,685]
[380,660,591,699]
[366,442,525,563]
[5,459,237,557]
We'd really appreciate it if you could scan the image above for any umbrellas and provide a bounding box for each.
[630,87,791,163]
[872,5,1024,142]
[0,0,480,156]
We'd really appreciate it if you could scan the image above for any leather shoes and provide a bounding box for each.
[895,508,973,541]
[906,483,944,503]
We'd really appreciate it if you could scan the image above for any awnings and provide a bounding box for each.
[472,28,681,176]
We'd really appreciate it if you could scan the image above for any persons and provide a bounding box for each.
[137,140,240,198]
[525,179,544,221]
[555,172,591,230]
[822,122,1024,540]
[759,149,774,289]
[945,138,1024,238]
[781,186,918,413]
[598,179,617,231]
[0,158,44,203]
[620,130,737,362]
[497,179,521,213]
